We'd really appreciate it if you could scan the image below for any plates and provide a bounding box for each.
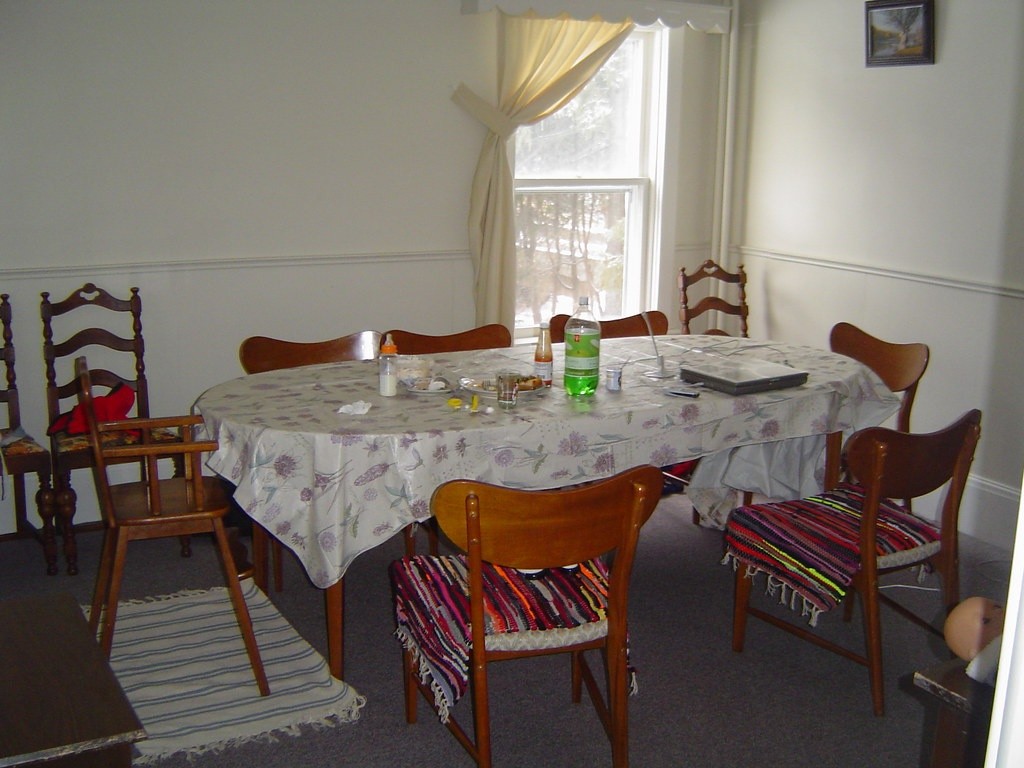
[460,376,543,397]
[406,385,455,395]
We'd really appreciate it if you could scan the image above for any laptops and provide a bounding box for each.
[680,357,809,397]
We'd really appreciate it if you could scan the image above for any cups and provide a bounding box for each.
[606,366,622,392]
[496,370,519,408]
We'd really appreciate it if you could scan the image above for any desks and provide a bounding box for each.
[194,335,907,678]
[0,592,150,768]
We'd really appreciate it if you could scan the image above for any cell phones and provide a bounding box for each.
[667,387,700,398]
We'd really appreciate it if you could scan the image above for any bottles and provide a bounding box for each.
[379,333,398,397]
[564,298,601,396]
[534,322,554,387]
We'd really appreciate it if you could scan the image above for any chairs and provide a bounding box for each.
[74,354,273,701]
[390,463,664,768]
[0,292,61,576]
[40,283,194,577]
[549,312,706,527]
[379,322,514,557]
[829,322,930,511]
[679,262,750,341]
[238,330,417,593]
[723,407,982,717]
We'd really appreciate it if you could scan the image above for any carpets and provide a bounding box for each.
[80,578,368,767]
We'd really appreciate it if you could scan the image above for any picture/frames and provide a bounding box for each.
[864,0,936,70]
